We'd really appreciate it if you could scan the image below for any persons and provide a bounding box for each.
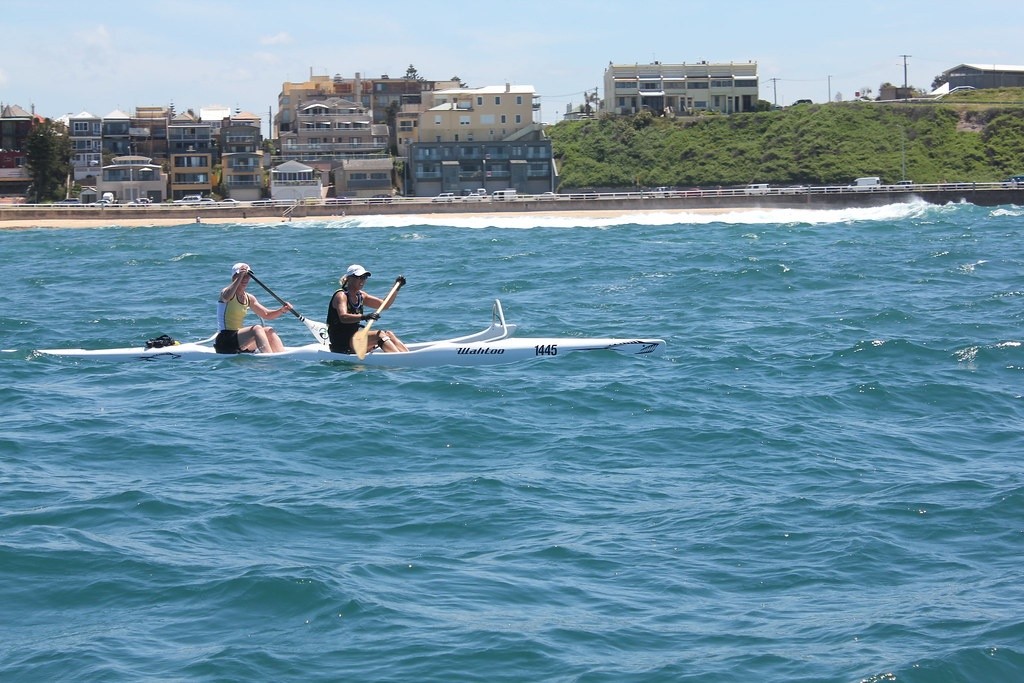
[326,264,410,353]
[213,263,292,354]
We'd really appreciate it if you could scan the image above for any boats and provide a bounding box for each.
[34,298,668,369]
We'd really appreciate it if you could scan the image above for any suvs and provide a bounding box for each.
[1002,175,1024,189]
[649,187,670,197]
[431,192,455,203]
[365,194,392,204]
[891,180,915,191]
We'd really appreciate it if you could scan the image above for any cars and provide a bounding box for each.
[781,185,806,195]
[0,192,320,208]
[533,191,560,200]
[942,182,968,190]
[679,188,705,196]
[325,196,352,205]
[463,194,482,202]
[570,190,599,200]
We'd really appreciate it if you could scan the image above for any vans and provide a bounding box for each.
[744,184,771,195]
[488,190,517,201]
[847,176,881,192]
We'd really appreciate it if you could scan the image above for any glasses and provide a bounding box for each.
[349,274,367,280]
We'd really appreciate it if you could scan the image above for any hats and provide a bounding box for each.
[346,264,371,277]
[232,263,254,277]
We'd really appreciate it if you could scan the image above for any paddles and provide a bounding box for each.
[247,271,332,346]
[349,279,402,361]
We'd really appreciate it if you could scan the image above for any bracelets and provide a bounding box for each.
[280,310,287,313]
